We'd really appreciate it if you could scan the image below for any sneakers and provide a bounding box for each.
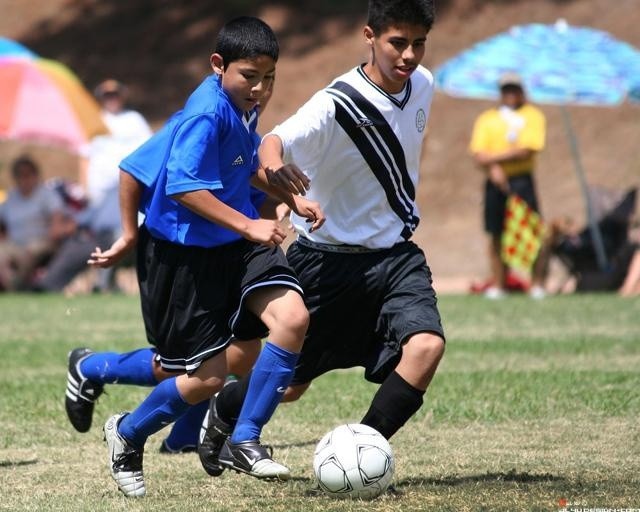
[64,346,104,434]
[217,435,292,481]
[103,412,146,499]
[198,379,239,477]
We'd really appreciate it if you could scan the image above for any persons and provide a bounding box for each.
[64,73,296,454]
[78,79,154,294]
[102,15,325,501]
[468,72,547,300]
[0,156,67,291]
[197,0,447,497]
[34,179,139,297]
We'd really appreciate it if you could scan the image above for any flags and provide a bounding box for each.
[500,191,547,277]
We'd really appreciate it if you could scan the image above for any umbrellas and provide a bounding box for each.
[435,17,640,271]
[0,38,111,158]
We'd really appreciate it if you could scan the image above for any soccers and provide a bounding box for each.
[312,423,395,501]
